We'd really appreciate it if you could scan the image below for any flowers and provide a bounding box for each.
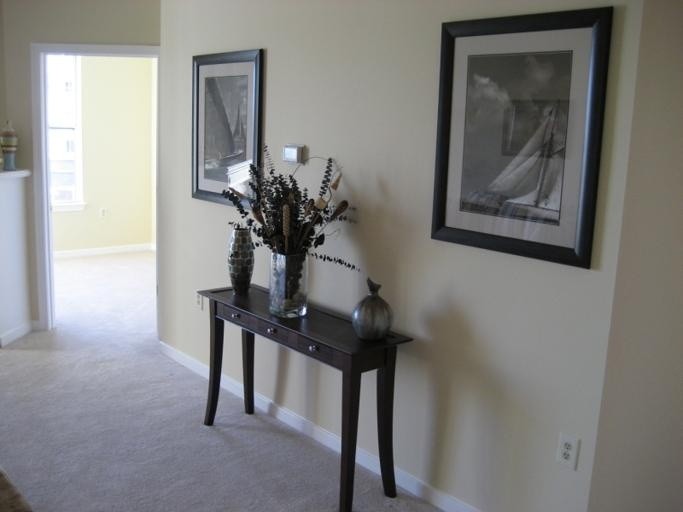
[221,141,362,307]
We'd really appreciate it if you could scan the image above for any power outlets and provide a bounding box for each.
[555,432,581,472]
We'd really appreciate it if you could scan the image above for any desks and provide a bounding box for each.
[197,283,414,512]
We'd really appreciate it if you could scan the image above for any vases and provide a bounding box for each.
[226,228,255,296]
[350,277,393,342]
[269,252,309,319]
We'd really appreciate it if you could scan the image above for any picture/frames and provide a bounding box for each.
[192,47,264,210]
[430,6,614,269]
